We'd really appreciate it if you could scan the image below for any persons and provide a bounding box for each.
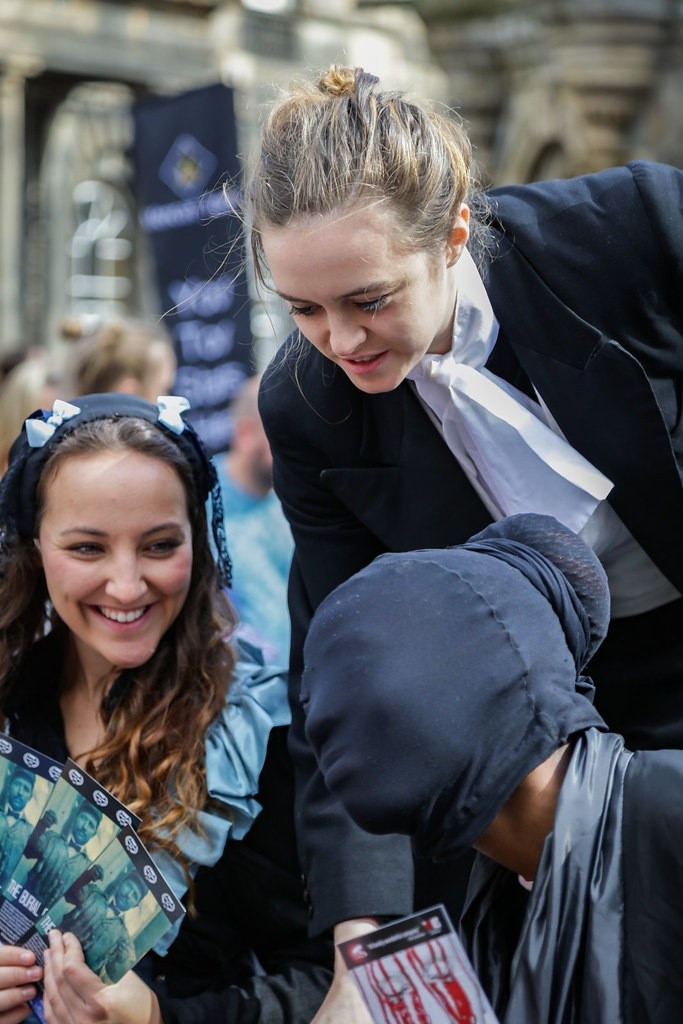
[245,61,683,1024]
[297,511,683,1024]
[0,317,296,727]
[0,392,335,1024]
